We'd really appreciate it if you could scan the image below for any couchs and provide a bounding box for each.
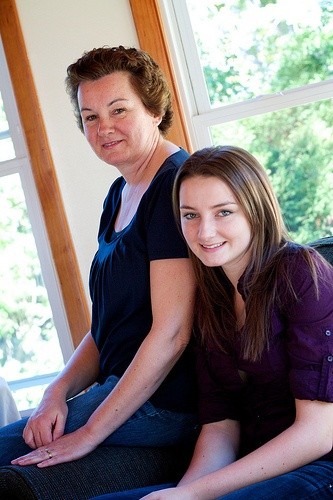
[0,235,333,499]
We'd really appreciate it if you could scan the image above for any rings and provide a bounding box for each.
[45,449,52,459]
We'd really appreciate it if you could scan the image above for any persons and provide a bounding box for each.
[0,45,196,500]
[142,145,333,500]
